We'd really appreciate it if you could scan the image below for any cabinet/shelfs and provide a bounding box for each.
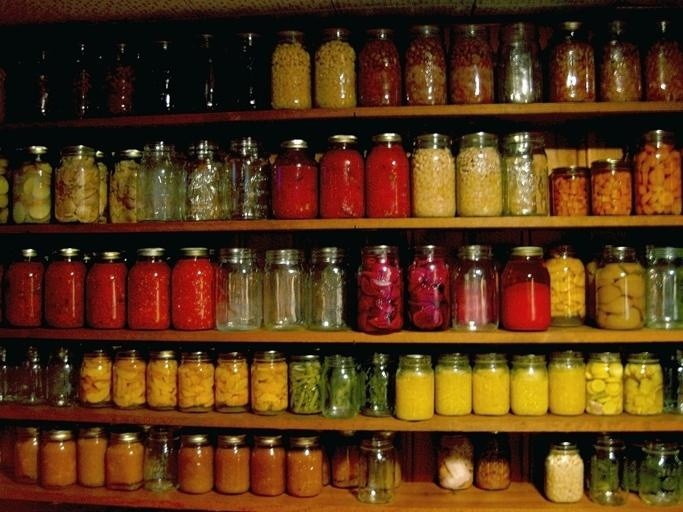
[1,1,682,511]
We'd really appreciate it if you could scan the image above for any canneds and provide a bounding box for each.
[434,353,473,415]
[395,354,436,420]
[319,358,361,419]
[593,241,645,329]
[303,248,353,331]
[477,431,513,493]
[438,431,474,490]
[217,246,264,332]
[357,245,406,333]
[270,30,312,110]
[214,436,249,495]
[402,26,448,105]
[544,440,585,504]
[11,146,52,223]
[264,249,311,330]
[358,438,394,504]
[1,31,267,120]
[53,145,100,222]
[170,146,196,221]
[95,150,108,217]
[288,355,324,414]
[109,148,144,223]
[251,350,288,413]
[287,436,322,498]
[6,247,45,328]
[550,351,588,415]
[406,246,452,330]
[588,352,625,415]
[45,247,87,329]
[510,356,550,417]
[646,248,683,330]
[142,144,178,220]
[625,353,665,413]
[214,354,249,411]
[16,426,39,485]
[169,246,217,330]
[585,437,627,505]
[46,349,76,405]
[249,436,286,498]
[448,24,495,103]
[144,427,179,491]
[75,428,108,488]
[544,246,586,328]
[21,349,46,404]
[356,29,403,107]
[333,437,360,488]
[320,133,366,218]
[313,29,356,109]
[361,354,395,418]
[179,433,214,493]
[456,131,503,216]
[224,136,272,220]
[411,133,456,217]
[451,245,501,333]
[642,20,683,102]
[637,441,681,505]
[188,144,223,220]
[108,433,144,489]
[634,128,683,214]
[503,132,550,216]
[591,160,634,215]
[177,352,214,412]
[128,248,170,329]
[40,429,76,487]
[665,352,683,415]
[0,347,20,402]
[145,351,176,408]
[620,440,644,494]
[551,166,591,216]
[1,147,11,224]
[500,247,551,330]
[114,350,145,408]
[76,348,113,408]
[273,139,320,219]
[473,354,510,415]
[495,23,542,104]
[600,20,642,101]
[541,22,597,102]
[366,133,411,218]
[86,249,127,329]
